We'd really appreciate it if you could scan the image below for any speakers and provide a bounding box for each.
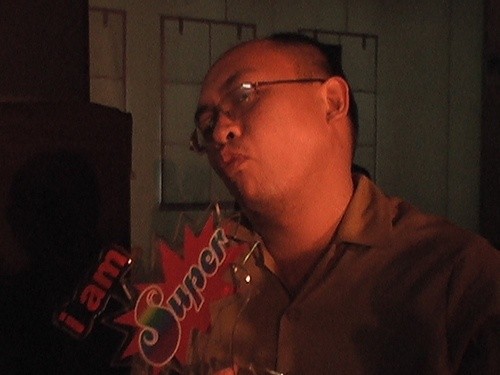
[0,95,132,375]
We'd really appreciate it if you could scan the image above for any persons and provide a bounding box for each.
[129,32,500,375]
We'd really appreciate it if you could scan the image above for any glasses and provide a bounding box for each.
[189,77,324,154]
